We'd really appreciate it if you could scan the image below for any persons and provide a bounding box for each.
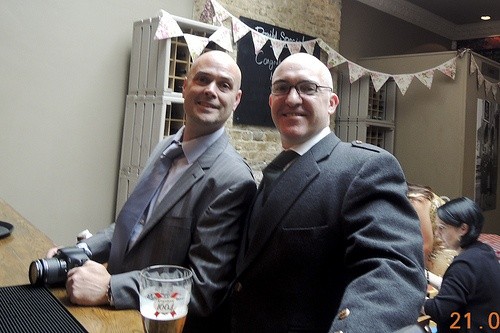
[421,197,500,333]
[45,50,258,318]
[182,52,428,333]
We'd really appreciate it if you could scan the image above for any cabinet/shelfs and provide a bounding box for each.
[337,74,397,156]
[114,17,231,223]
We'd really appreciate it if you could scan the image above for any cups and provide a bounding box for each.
[139,266,193,333]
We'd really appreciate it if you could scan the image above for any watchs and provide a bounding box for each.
[427,270,430,285]
[106,278,112,302]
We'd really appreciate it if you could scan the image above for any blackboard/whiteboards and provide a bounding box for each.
[233,16,319,129]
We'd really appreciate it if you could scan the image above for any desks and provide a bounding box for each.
[0,197,146,333]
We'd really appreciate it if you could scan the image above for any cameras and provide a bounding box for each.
[29,245,92,289]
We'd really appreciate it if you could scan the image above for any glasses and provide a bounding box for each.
[269,80,332,96]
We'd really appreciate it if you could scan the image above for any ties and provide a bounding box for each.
[248,150,300,248]
[108,142,182,271]
